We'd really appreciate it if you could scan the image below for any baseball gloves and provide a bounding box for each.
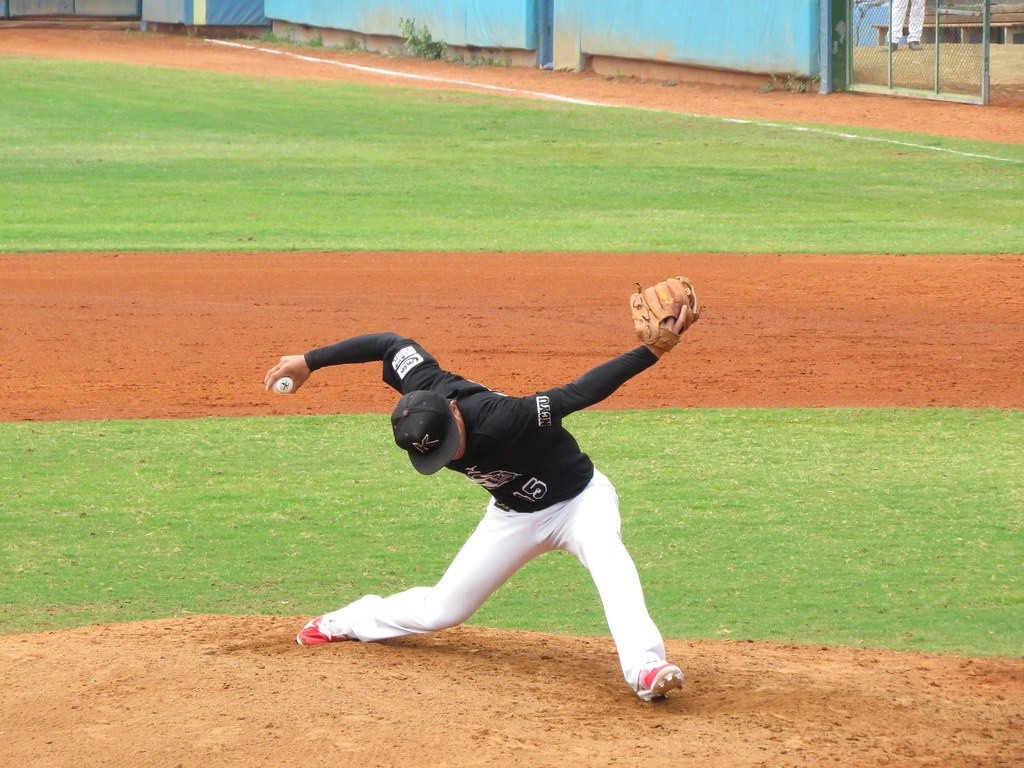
[629,275,700,353]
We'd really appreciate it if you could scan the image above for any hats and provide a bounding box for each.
[390,390,460,475]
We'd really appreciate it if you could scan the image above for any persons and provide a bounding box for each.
[260,275,702,701]
[888,1,924,49]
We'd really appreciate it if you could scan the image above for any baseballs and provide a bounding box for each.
[272,376,293,395]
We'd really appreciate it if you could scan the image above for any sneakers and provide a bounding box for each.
[634,660,684,703]
[295,608,352,645]
